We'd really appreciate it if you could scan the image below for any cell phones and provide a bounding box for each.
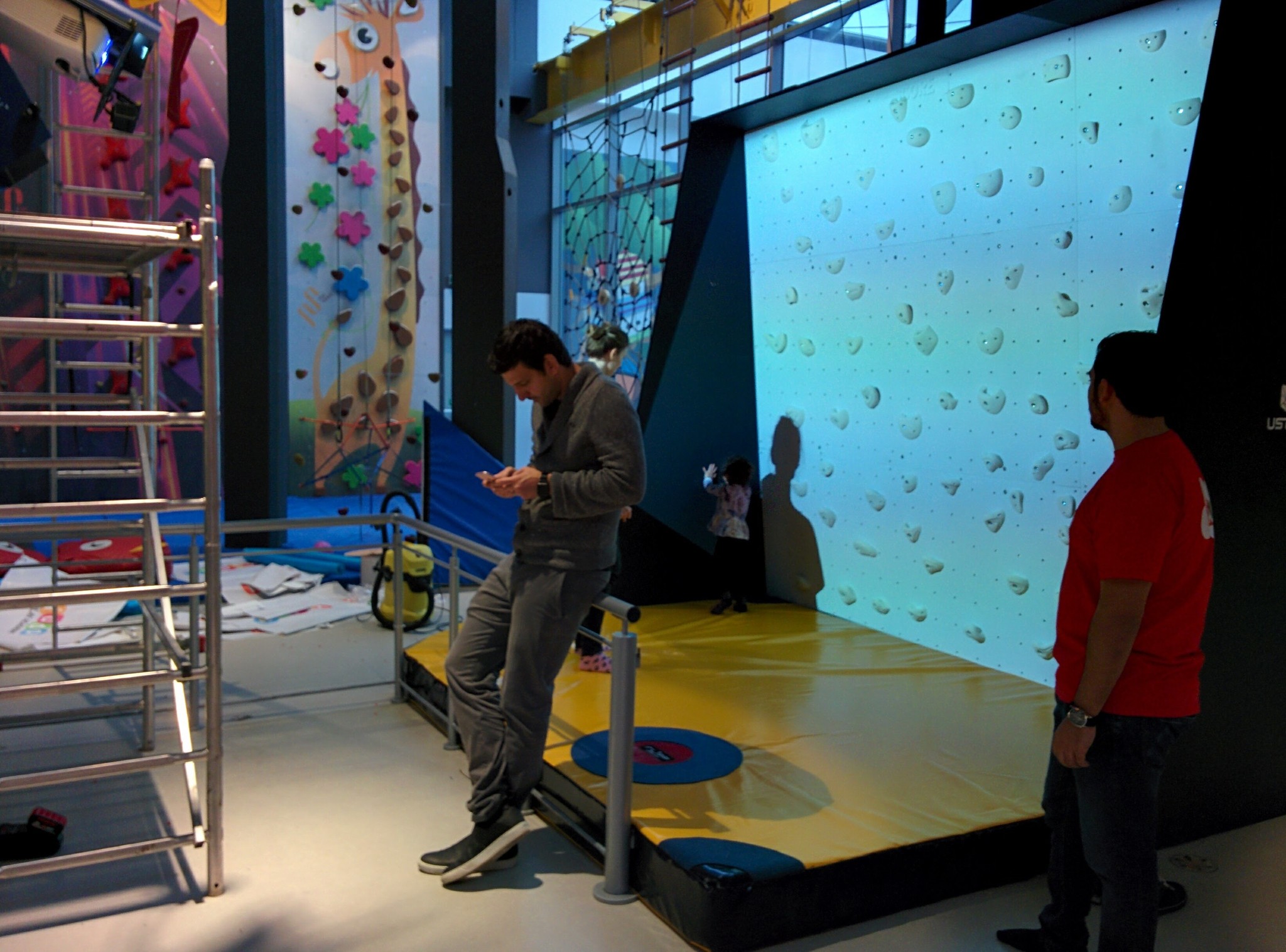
[475,470,508,488]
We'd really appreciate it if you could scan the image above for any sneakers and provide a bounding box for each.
[441,807,529,884]
[418,829,520,875]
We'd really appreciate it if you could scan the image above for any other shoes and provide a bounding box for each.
[1157,880,1187,915]
[997,927,1087,952]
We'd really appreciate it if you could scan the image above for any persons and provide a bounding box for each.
[994,332,1214,951]
[416,319,647,885]
[705,457,753,614]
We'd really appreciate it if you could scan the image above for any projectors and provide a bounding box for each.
[0,0,112,81]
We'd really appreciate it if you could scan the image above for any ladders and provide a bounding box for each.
[43,44,156,650]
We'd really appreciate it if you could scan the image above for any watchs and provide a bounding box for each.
[1065,702,1097,728]
